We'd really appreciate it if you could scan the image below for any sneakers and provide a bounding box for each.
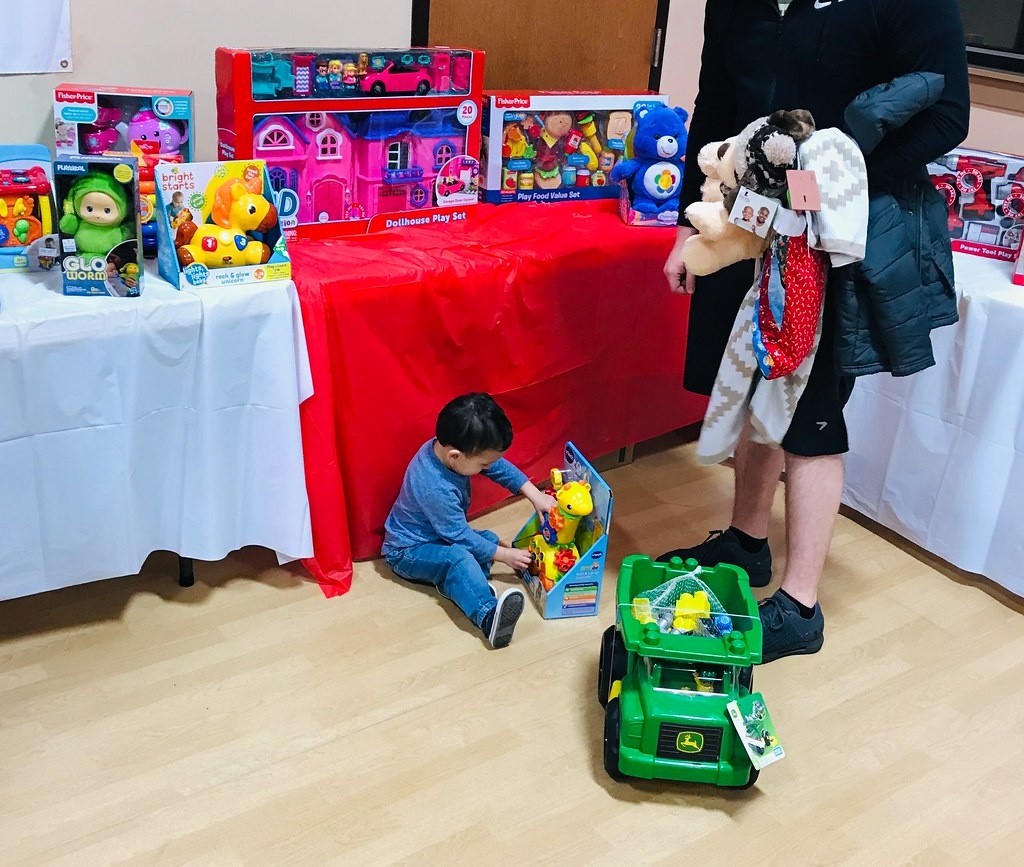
[483,587,525,649]
[436,582,497,602]
[757,590,824,664]
[654,529,772,587]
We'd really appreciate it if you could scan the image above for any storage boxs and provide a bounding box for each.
[926,147,1024,285]
[53,154,144,297]
[479,89,669,204]
[214,48,487,242]
[52,84,197,259]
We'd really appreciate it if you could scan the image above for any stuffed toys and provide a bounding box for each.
[682,110,813,276]
[612,106,688,212]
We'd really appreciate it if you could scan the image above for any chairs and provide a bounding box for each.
[275,62,296,91]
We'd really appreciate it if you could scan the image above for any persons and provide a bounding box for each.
[59,170,136,263]
[166,191,189,222]
[316,60,357,89]
[654,0,971,662]
[734,206,769,237]
[106,262,130,297]
[380,392,558,649]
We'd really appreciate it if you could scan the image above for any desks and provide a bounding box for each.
[285,198,713,598]
[0,257,314,603]
[730,252,1024,599]
